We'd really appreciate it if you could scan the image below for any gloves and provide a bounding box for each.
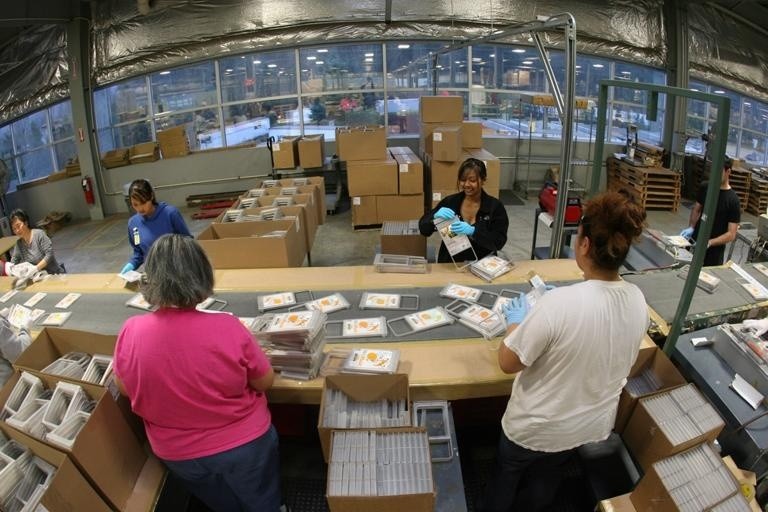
[502,292,527,326]
[5,262,14,275]
[680,228,692,240]
[450,221,475,235]
[434,207,455,220]
[19,261,41,276]
[121,262,134,275]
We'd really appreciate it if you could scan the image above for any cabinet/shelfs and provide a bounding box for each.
[530,207,576,259]
[513,98,593,200]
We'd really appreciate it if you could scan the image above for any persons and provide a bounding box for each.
[682,153,742,266]
[130,74,375,141]
[120,179,191,273]
[494,190,650,510]
[419,158,508,263]
[113,231,285,512]
[9,209,64,287]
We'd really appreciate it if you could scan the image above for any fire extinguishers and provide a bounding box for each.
[81,175,94,205]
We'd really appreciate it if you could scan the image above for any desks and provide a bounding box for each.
[0,236,21,256]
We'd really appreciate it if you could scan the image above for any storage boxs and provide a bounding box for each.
[629,439,754,512]
[347,146,425,224]
[272,135,302,169]
[419,96,463,123]
[422,122,482,148]
[0,368,148,512]
[621,383,725,474]
[12,327,148,447]
[380,220,426,260]
[0,421,114,512]
[298,134,325,168]
[326,427,434,512]
[195,177,326,269]
[433,125,463,162]
[335,125,386,162]
[317,374,412,464]
[613,346,688,432]
[419,145,500,210]
